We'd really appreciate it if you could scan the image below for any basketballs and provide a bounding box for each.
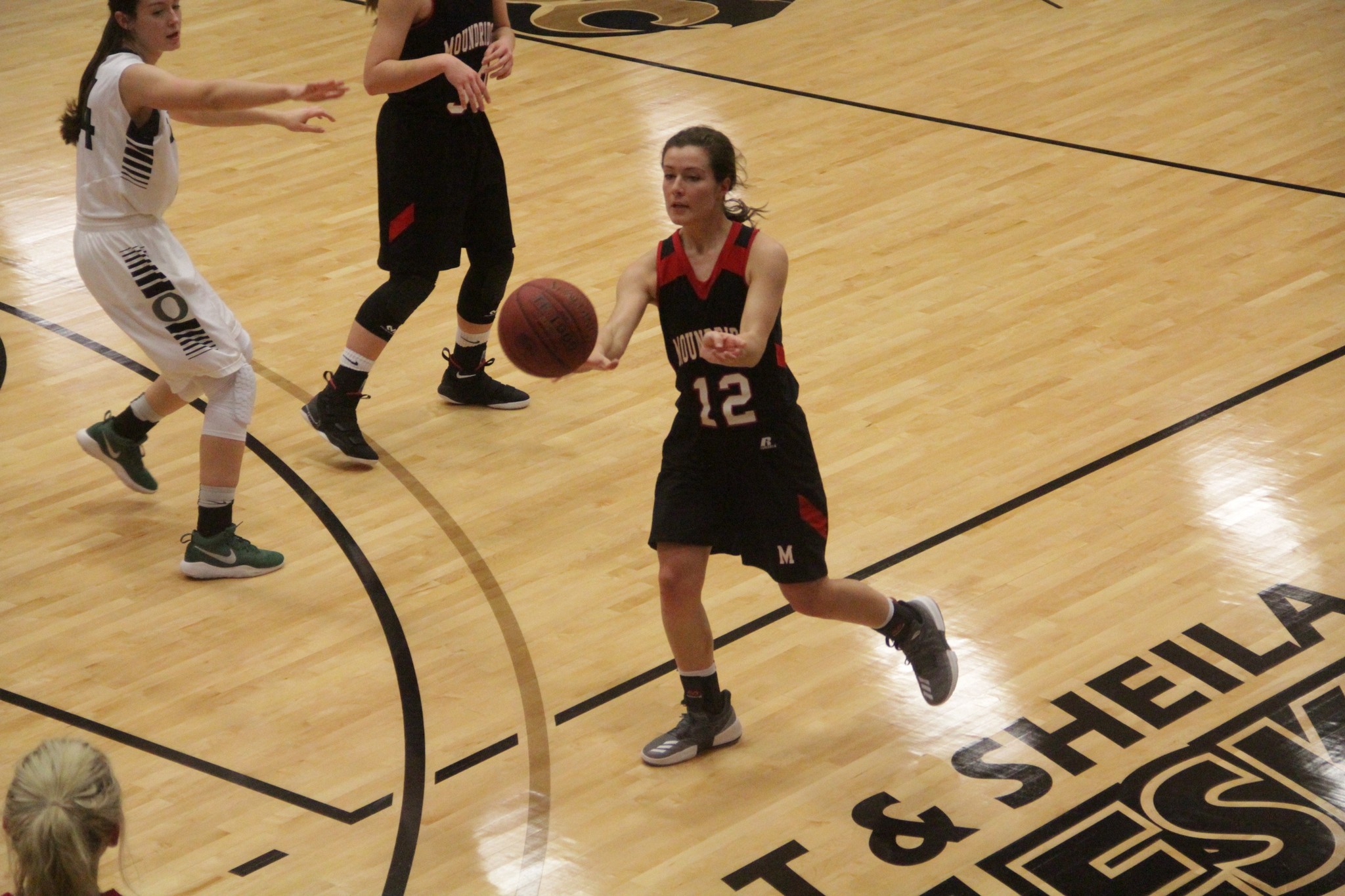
[498,277,597,376]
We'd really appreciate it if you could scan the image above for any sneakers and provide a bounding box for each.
[886,596,958,706]
[642,690,741,765]
[438,347,530,409]
[181,521,285,578]
[301,371,378,465]
[77,410,158,494]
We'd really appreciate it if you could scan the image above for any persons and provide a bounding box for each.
[59,0,352,578]
[298,0,529,463]
[552,127,959,764]
[0,739,122,896]
